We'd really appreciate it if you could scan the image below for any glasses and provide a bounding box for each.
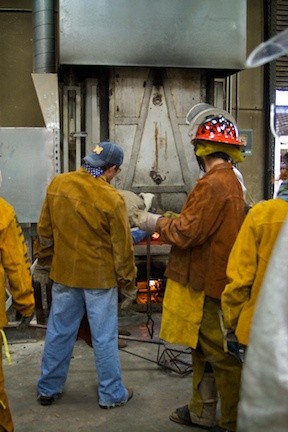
[117,167,121,173]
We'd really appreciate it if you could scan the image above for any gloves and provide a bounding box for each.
[17,314,34,330]
[132,212,164,233]
[120,287,137,309]
[30,258,49,284]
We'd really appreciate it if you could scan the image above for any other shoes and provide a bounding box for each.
[39,396,53,405]
[170,405,212,430]
[99,387,133,409]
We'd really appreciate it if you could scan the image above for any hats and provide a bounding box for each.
[82,142,124,167]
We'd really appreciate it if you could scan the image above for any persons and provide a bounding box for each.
[34,142,134,410]
[217,152,288,363]
[0,198,35,432]
[236,25,288,432]
[134,103,249,432]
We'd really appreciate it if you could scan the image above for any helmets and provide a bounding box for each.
[191,114,244,148]
